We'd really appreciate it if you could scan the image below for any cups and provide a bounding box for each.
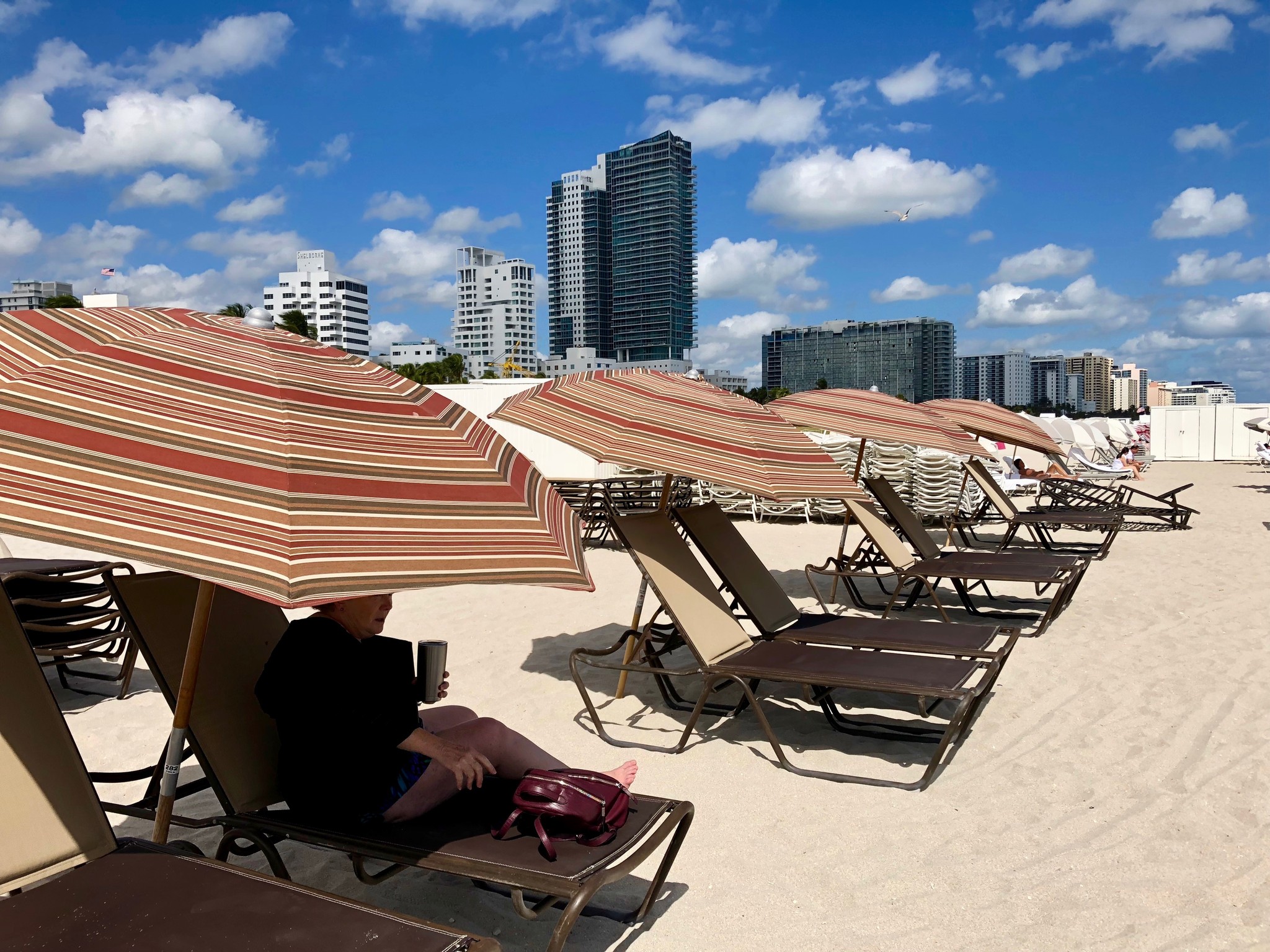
[418,639,448,702]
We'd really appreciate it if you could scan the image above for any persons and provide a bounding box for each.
[1125,445,1145,479]
[1264,443,1270,449]
[984,458,1050,481]
[1112,447,1145,481]
[1014,458,1081,480]
[254,592,639,824]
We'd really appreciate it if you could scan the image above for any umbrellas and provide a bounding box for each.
[1007,409,1140,480]
[916,398,1068,547]
[762,385,1001,604]
[0,306,597,843]
[1244,417,1270,445]
[488,369,877,701]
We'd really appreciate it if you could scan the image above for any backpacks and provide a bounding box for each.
[491,769,638,859]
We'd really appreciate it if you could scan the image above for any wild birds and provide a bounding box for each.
[883,203,925,222]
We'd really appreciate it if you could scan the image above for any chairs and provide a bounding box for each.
[0,436,1200,952]
[1253,441,1270,472]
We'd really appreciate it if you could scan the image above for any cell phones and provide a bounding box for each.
[1128,462,1132,464]
[1143,462,1146,463]
